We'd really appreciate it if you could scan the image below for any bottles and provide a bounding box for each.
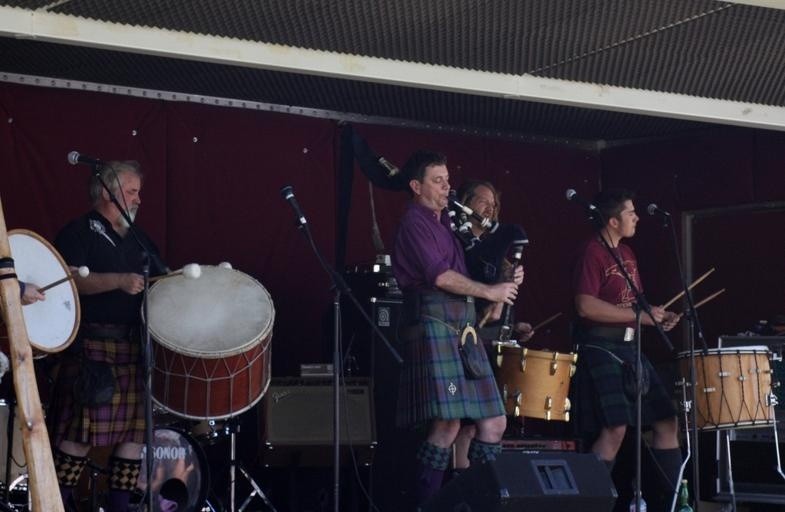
[677,479,694,512]
[629,490,647,512]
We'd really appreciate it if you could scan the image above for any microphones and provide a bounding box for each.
[67,151,107,167]
[648,202,674,220]
[283,185,307,226]
[565,187,602,217]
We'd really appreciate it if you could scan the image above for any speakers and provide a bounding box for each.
[428,447,618,512]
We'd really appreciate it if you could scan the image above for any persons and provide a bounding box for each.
[570,189,686,512]
[52,159,175,511]
[0,279,46,510]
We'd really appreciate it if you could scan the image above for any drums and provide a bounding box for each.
[489,340,578,423]
[137,426,211,512]
[674,345,779,434]
[0,400,47,491]
[0,228,81,360]
[140,264,276,421]
